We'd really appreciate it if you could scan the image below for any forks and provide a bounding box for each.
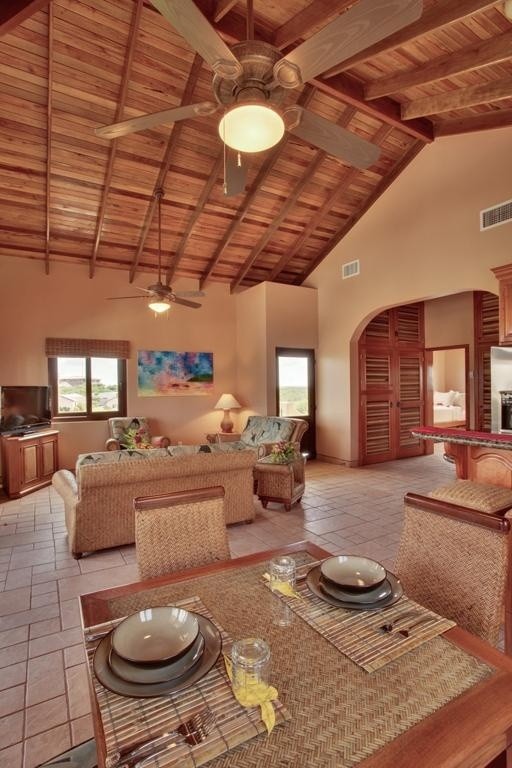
[127,714,219,767]
[104,704,212,766]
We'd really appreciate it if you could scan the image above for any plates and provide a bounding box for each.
[308,565,405,612]
[94,606,223,699]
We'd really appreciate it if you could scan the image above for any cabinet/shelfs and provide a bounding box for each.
[1,434,58,493]
[499,278,512,346]
[359,304,424,464]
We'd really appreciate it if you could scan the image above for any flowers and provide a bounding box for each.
[120,427,150,450]
[270,437,300,463]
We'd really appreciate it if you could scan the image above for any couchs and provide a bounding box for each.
[50,448,259,560]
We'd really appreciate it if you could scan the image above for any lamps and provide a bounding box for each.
[213,392,243,434]
[218,85,286,157]
[148,299,171,314]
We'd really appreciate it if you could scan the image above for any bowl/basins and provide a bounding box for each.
[319,555,389,593]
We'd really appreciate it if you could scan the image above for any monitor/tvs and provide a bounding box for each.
[0,385,53,437]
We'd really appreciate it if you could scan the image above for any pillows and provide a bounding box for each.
[433,389,465,406]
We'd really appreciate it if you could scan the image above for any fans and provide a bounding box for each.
[103,189,206,309]
[92,0,430,200]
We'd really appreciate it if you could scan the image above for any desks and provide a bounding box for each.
[206,433,240,443]
[80,538,512,768]
[254,451,311,512]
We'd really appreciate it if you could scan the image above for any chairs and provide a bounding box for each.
[389,491,512,655]
[131,485,234,582]
[207,416,308,493]
[104,416,171,451]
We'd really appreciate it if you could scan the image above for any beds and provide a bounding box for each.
[433,392,466,423]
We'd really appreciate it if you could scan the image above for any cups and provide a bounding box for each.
[233,637,272,708]
[267,556,300,606]
[269,604,297,628]
